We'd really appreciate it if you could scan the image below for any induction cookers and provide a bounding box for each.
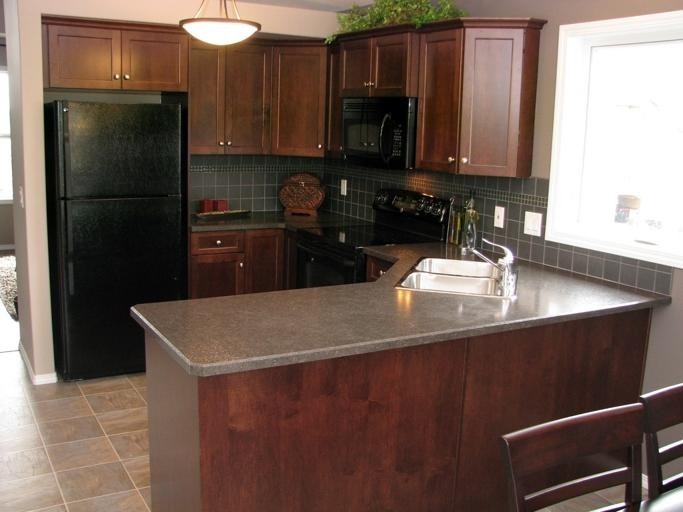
[298,222,442,252]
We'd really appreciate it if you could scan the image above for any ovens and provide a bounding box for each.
[296,244,368,288]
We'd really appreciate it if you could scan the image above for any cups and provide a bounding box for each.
[213,199,226,211]
[199,200,213,213]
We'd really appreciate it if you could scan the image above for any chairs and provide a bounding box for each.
[639,383,683,501]
[500,402,650,512]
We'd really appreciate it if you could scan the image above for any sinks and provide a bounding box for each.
[405,255,501,278]
[393,269,515,299]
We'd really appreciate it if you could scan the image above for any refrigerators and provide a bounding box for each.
[45,101,184,382]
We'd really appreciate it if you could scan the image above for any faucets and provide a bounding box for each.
[468,237,518,293]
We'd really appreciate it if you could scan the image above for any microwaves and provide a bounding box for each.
[342,96,415,169]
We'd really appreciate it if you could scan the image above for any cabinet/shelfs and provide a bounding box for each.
[245,228,285,294]
[42,14,189,94]
[188,33,273,155]
[415,17,548,177]
[272,40,329,158]
[190,230,246,299]
[328,22,420,159]
[366,255,395,282]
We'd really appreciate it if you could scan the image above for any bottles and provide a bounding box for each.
[460,188,477,250]
[446,193,465,245]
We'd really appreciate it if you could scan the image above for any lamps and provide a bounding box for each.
[179,1,262,47]
